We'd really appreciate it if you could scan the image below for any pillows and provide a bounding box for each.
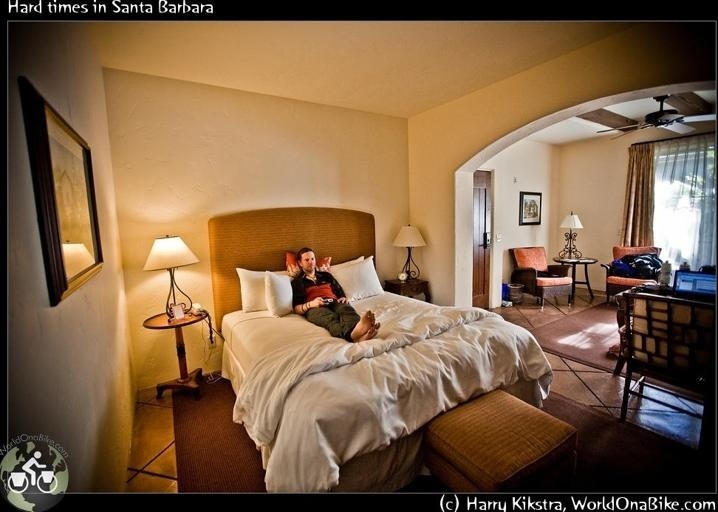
[236,252,385,318]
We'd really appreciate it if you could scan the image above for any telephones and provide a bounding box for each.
[190,303,205,316]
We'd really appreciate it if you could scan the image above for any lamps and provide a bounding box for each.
[391,223,428,279]
[558,211,583,258]
[143,234,201,321]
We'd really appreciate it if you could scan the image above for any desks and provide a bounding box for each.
[142,310,210,399]
[553,255,598,303]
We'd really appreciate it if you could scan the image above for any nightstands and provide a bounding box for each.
[385,279,430,301]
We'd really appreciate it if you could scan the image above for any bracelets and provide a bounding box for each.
[301,301,311,315]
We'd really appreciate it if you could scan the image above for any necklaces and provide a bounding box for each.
[306,274,315,280]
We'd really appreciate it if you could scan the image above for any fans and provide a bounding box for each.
[597,95,716,140]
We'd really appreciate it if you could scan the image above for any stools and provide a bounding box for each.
[425,389,577,491]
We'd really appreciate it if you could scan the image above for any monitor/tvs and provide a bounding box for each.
[673,270,717,295]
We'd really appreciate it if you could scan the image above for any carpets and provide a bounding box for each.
[173,370,715,498]
[528,301,706,404]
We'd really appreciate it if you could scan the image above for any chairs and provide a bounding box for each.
[600,245,662,302]
[510,247,574,311]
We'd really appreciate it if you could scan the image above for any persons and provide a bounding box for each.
[291,247,382,344]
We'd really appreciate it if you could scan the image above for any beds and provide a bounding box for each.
[221,292,552,493]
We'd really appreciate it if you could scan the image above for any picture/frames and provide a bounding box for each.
[21,78,106,306]
[520,191,542,225]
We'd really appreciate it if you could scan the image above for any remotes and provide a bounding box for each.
[321,298,333,303]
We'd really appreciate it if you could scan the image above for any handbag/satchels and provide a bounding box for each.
[629,255,662,279]
[612,259,632,277]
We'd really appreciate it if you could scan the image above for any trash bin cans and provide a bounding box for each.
[502,283,508,300]
[509,284,524,303]
